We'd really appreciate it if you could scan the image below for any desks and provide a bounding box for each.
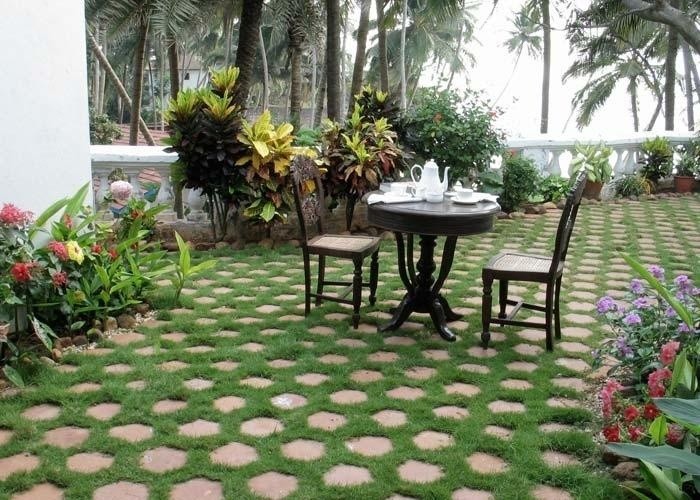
[367,193,501,341]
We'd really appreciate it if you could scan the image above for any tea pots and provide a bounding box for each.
[409,158,451,200]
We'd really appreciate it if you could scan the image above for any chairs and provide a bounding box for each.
[481,168,588,352]
[290,153,382,329]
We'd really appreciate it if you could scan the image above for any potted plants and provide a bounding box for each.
[500,158,537,213]
[568,140,615,199]
[674,129,700,193]
[637,136,676,192]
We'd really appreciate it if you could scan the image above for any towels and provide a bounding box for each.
[444,188,500,203]
[366,192,423,205]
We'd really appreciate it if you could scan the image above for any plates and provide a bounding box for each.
[449,196,479,206]
[445,190,458,196]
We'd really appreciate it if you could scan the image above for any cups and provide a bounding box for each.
[412,185,427,201]
[424,189,444,203]
[391,182,407,196]
[456,188,473,199]
